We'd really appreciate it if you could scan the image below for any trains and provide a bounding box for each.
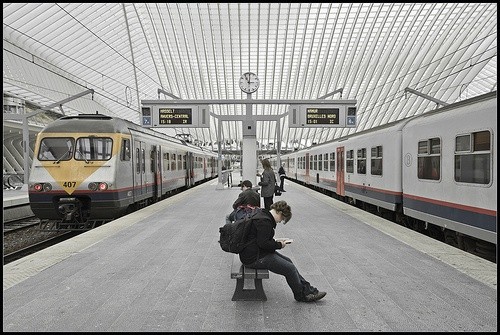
[27,111,225,231]
[266,92,498,245]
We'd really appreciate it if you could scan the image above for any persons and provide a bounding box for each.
[256,159,286,209]
[218,180,261,243]
[239,200,327,302]
[222,166,231,175]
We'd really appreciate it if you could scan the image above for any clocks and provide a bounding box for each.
[239,72,260,93]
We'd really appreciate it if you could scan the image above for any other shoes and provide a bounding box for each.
[305,292,326,302]
[282,190,286,192]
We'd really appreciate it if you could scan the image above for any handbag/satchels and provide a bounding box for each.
[275,179,281,196]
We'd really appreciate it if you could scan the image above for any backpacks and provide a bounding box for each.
[220,207,272,252]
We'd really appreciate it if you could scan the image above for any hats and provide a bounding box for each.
[238,180,252,188]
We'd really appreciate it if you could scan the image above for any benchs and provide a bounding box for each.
[230,254,269,302]
